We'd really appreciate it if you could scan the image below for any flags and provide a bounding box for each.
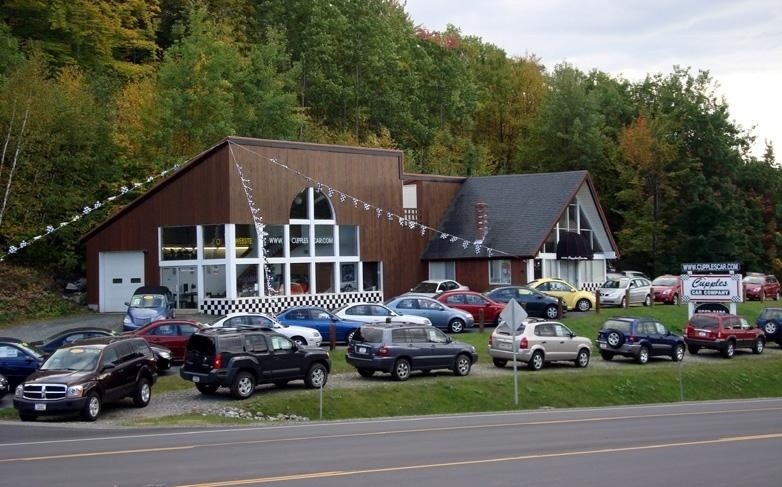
[0,164,179,260]
[271,157,494,257]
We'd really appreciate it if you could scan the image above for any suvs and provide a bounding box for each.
[688,313,766,356]
[489,317,593,368]
[758,308,782,349]
[0,336,46,393]
[180,328,332,399]
[598,314,685,361]
[123,286,176,332]
[346,322,479,382]
[15,335,157,421]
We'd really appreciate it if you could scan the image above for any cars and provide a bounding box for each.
[207,313,323,349]
[597,275,652,309]
[275,305,367,342]
[524,276,597,312]
[650,274,681,305]
[742,274,781,303]
[119,321,205,368]
[423,289,508,326]
[482,284,568,319]
[380,294,475,334]
[0,376,8,398]
[398,278,470,304]
[32,327,118,355]
[331,301,433,330]
